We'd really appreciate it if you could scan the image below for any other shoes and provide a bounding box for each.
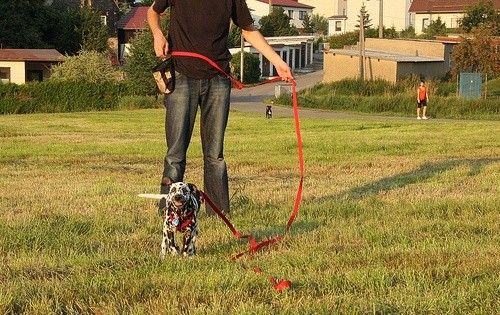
[422,117,427,119]
[417,117,421,119]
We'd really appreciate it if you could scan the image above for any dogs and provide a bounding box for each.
[266,106,273,119]
[160,181,204,257]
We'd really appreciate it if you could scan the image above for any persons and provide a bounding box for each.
[146,0,297,217]
[416,80,429,120]
